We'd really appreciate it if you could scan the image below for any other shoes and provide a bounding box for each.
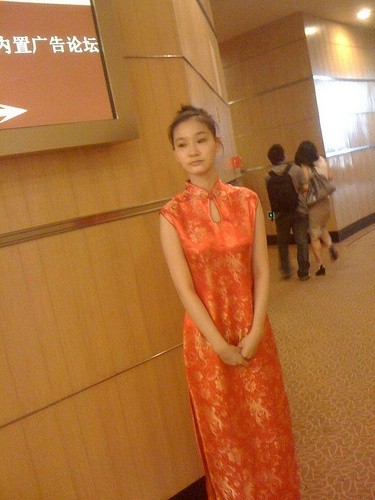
[315,265,326,276]
[329,246,339,260]
[281,272,292,280]
[301,274,310,281]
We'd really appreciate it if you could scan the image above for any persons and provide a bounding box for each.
[158,105,301,500]
[265,140,339,281]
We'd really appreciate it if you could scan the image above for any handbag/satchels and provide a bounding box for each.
[305,164,336,207]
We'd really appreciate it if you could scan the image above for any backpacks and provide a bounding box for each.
[267,163,298,213]
